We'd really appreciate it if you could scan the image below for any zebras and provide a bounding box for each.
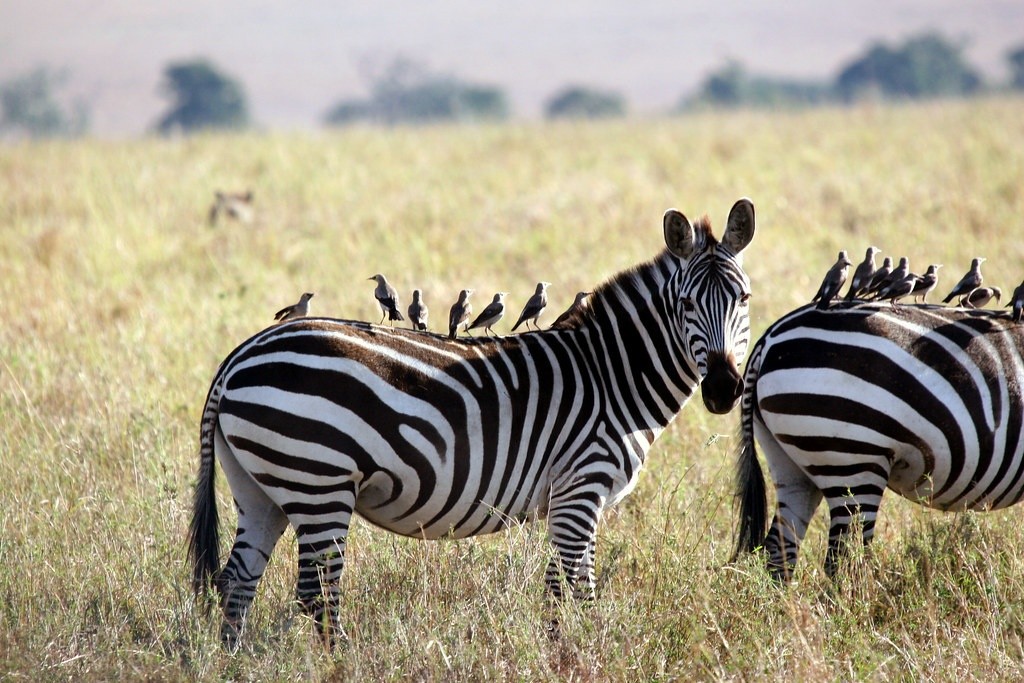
[733,302,1024,611]
[184,197,758,671]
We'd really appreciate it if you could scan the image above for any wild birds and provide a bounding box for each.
[448,289,510,339]
[511,281,552,333]
[809,247,942,305]
[408,290,430,333]
[367,274,406,329]
[553,292,594,326]
[942,257,1024,321]
[274,293,314,323]
